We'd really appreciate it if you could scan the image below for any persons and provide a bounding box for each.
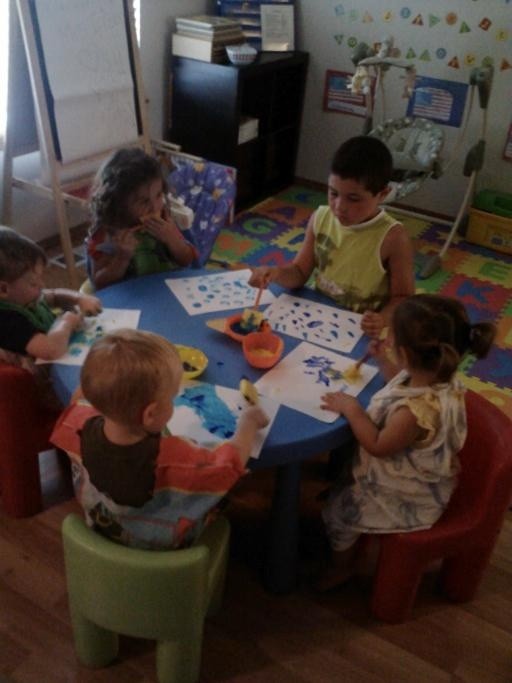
[320,293,497,592]
[1,226,104,417]
[248,135,415,340]
[49,329,271,551]
[78,146,197,294]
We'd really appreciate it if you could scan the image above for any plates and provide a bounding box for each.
[224,313,271,343]
[171,342,209,380]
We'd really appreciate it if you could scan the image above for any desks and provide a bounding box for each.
[47,269,386,595]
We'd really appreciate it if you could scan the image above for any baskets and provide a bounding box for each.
[225,45,257,66]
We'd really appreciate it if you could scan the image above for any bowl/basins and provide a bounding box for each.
[243,333,284,369]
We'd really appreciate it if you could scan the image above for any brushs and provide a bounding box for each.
[240,281,264,331]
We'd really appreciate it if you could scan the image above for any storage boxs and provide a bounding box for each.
[464,204,512,255]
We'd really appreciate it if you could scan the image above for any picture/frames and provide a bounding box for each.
[323,68,378,119]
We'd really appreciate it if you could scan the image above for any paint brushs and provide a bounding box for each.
[342,338,385,378]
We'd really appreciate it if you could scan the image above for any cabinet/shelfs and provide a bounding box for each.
[167,45,310,216]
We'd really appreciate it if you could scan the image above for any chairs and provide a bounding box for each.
[59,509,231,683]
[359,385,512,622]
[349,51,496,276]
[1,358,74,517]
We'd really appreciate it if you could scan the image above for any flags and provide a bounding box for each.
[326,75,365,104]
[412,87,455,122]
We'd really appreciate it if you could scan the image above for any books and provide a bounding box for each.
[259,3,295,53]
[170,14,245,64]
